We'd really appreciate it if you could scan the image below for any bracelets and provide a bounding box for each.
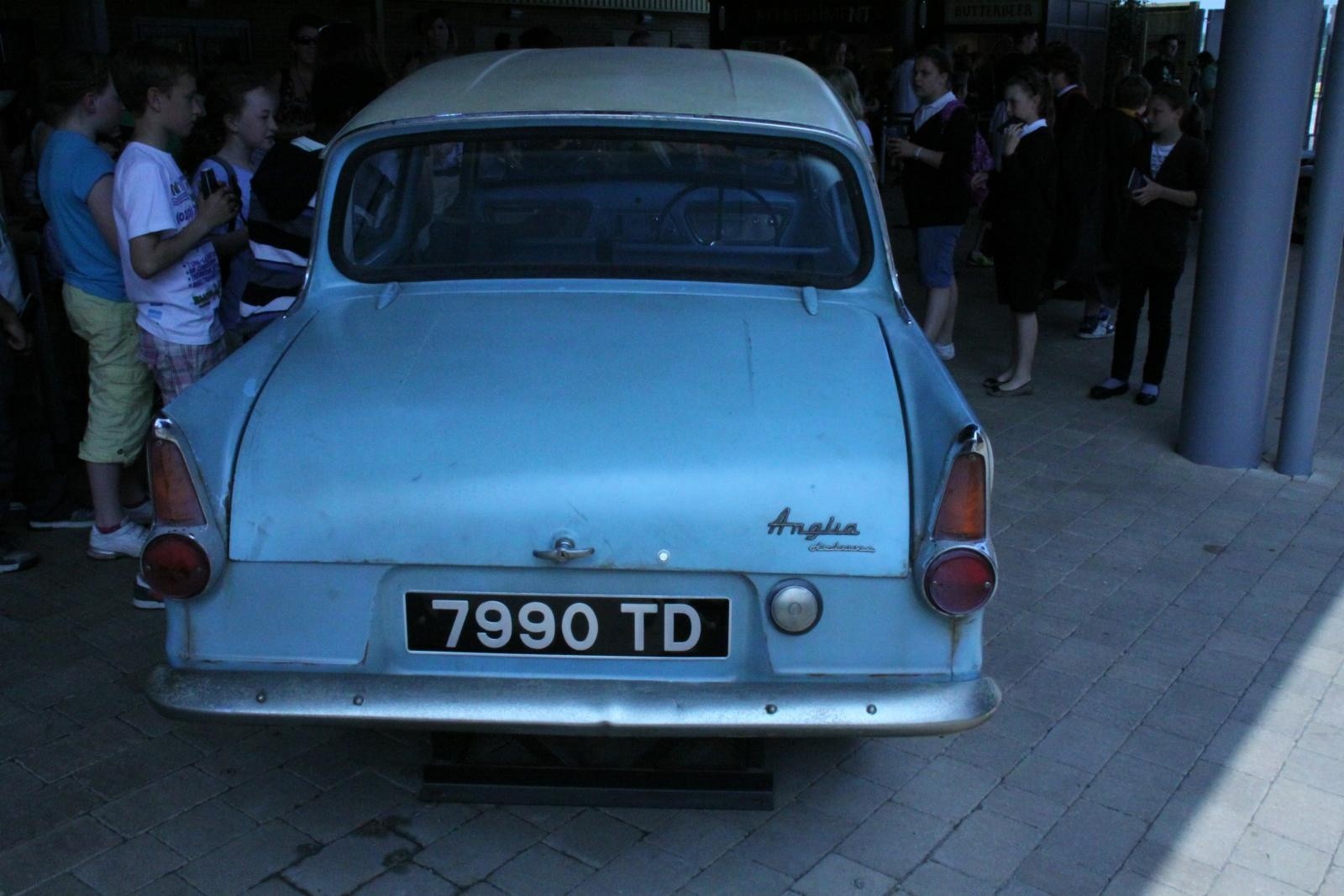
[915,146,923,160]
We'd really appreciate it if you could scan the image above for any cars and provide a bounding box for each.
[135,47,1003,740]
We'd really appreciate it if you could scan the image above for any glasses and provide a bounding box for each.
[294,35,317,45]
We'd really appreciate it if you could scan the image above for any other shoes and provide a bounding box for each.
[966,249,994,267]
[0,541,39,573]
[933,342,955,361]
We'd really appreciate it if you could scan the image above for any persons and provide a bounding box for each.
[1090,84,1210,405]
[888,49,976,361]
[772,20,1217,302]
[111,54,242,610]
[0,293,41,576]
[233,79,369,343]
[2,11,564,134]
[39,50,156,560]
[1070,75,1153,340]
[187,73,278,356]
[970,74,1056,398]
[818,67,873,158]
[3,145,96,529]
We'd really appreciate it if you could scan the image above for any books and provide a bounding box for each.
[1126,166,1146,197]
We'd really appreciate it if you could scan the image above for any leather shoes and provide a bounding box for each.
[1136,383,1160,405]
[1091,377,1129,399]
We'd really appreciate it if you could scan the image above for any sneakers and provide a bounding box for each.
[1077,321,1117,339]
[25,500,96,529]
[121,489,152,523]
[1097,308,1111,325]
[87,518,150,560]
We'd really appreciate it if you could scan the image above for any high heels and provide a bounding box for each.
[987,378,1034,397]
[982,376,1011,388]
